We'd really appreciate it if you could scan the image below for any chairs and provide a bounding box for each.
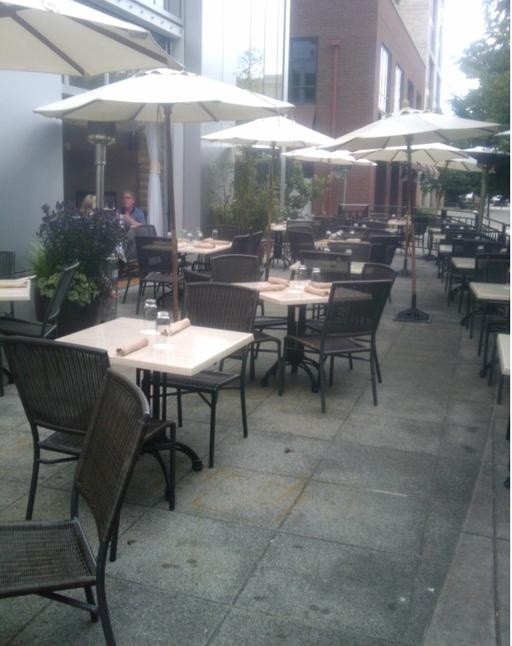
[2,331,173,561]
[2,366,153,645]
[424,204,510,401]
[0,210,411,513]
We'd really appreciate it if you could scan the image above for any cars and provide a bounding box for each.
[493,194,506,205]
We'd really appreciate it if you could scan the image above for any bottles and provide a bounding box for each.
[144,298,158,331]
[155,311,172,349]
[294,264,308,290]
[311,268,320,289]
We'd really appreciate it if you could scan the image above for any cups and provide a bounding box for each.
[168,227,219,250]
